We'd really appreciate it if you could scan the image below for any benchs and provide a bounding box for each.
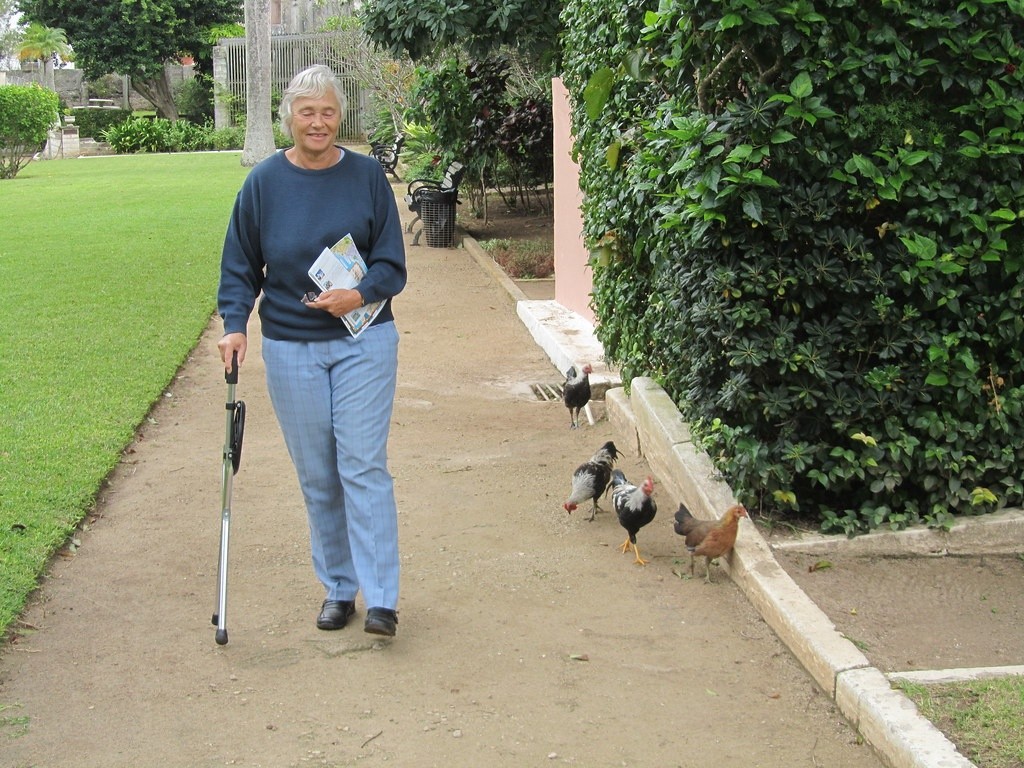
[367,133,405,183]
[402,161,469,246]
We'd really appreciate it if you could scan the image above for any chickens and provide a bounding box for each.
[562,363,593,430]
[605,469,658,567]
[564,440,626,522]
[673,501,748,585]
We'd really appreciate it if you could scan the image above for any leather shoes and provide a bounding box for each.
[365,605,398,636]
[317,599,355,629]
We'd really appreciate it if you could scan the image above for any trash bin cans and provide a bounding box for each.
[421,188,457,248]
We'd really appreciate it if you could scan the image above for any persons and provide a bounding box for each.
[217,64,408,636]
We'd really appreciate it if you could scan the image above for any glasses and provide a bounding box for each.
[301,290,319,304]
